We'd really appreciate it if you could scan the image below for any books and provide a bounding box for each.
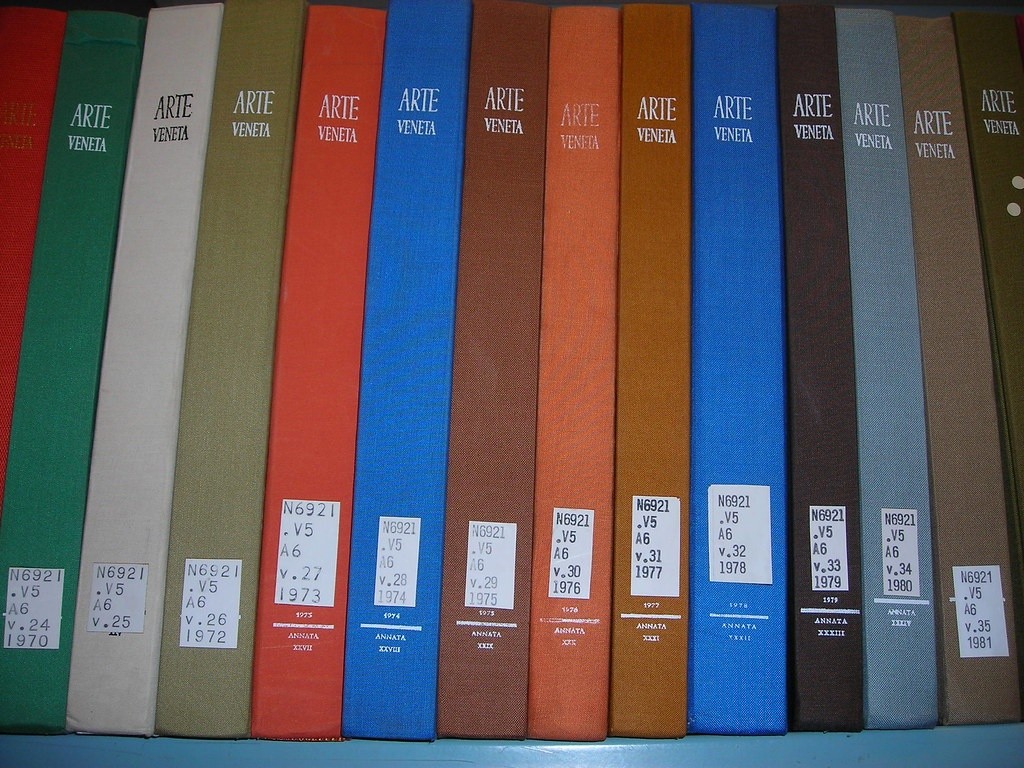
[0,2,1023,744]
